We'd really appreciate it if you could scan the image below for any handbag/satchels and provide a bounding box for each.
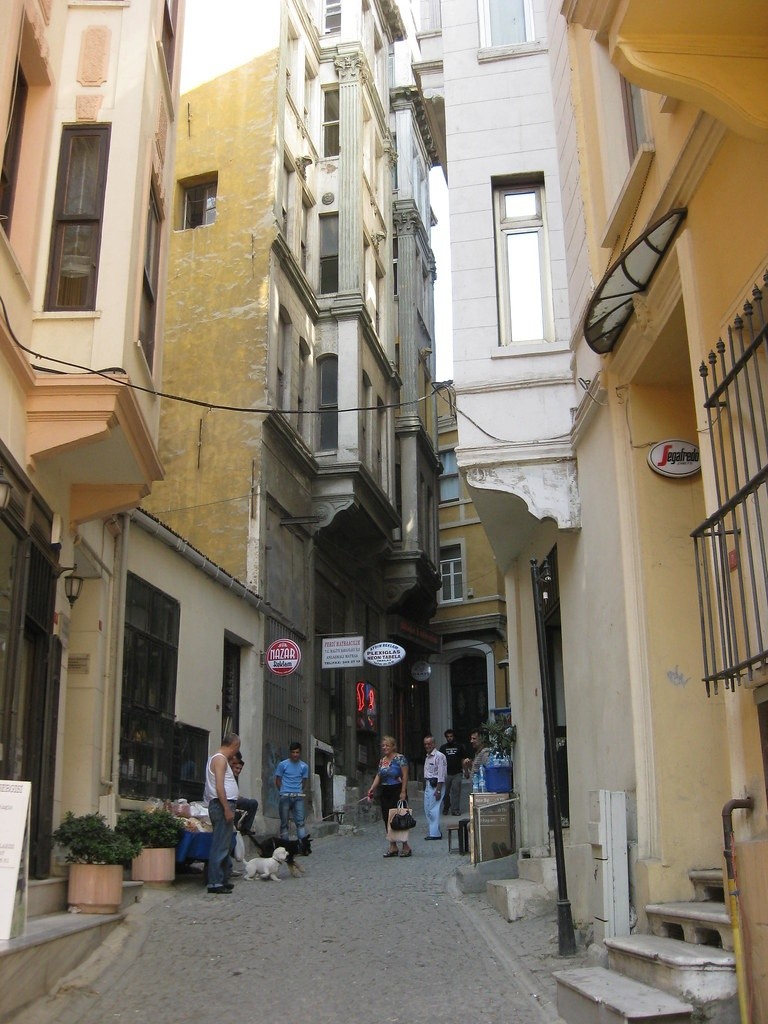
[386,799,413,841]
[234,830,247,862]
[427,778,438,787]
[389,800,417,830]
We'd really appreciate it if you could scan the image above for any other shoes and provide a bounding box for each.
[224,883,235,890]
[443,807,449,815]
[451,809,461,816]
[424,835,442,840]
[207,885,233,894]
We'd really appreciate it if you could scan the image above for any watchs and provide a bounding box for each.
[436,790,441,794]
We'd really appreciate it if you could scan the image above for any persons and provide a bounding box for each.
[438,729,470,816]
[367,736,412,858]
[470,728,493,777]
[275,742,309,842]
[423,735,447,840]
[202,732,258,894]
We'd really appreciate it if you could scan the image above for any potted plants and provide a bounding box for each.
[116,807,185,886]
[482,714,513,796]
[52,809,141,917]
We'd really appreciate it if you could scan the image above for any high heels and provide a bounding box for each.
[383,849,399,856]
[400,848,412,857]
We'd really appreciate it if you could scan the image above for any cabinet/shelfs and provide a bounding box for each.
[120,704,177,801]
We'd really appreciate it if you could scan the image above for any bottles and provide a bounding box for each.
[472,764,486,793]
[488,753,512,768]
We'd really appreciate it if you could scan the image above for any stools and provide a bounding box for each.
[447,823,460,852]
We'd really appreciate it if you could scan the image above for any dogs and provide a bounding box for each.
[242,833,314,882]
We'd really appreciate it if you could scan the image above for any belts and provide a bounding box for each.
[283,792,307,797]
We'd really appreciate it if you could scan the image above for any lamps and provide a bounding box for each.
[61,564,85,610]
[0,463,14,515]
[467,587,475,599]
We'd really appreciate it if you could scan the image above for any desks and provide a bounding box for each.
[176,828,238,872]
